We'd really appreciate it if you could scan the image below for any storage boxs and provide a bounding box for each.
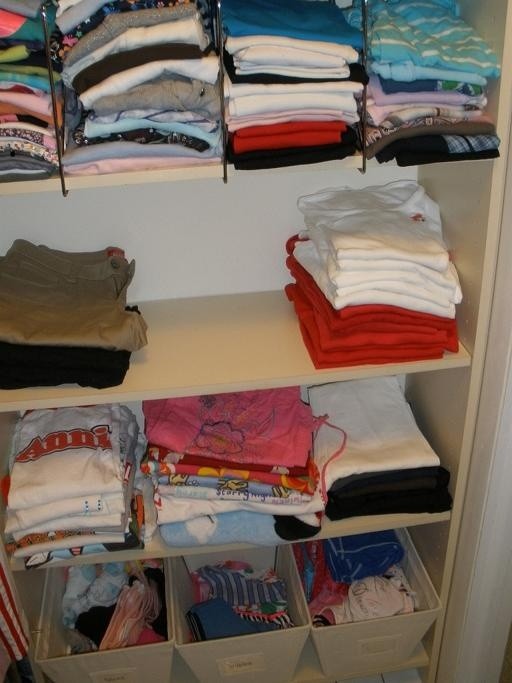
[33,558,176,683]
[168,544,312,683]
[291,526,443,676]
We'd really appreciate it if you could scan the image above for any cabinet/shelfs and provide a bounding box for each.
[0,1,511,682]
